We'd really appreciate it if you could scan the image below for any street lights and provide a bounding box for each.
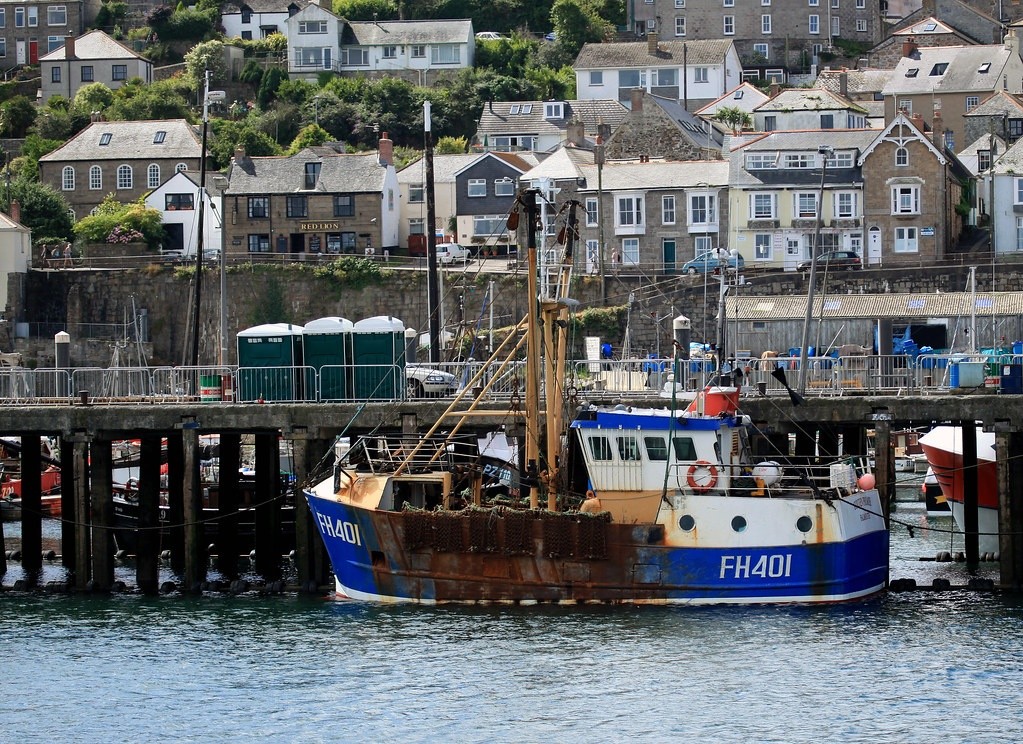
[798,145,835,394]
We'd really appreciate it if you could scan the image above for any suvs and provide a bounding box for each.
[796,250,862,271]
[682,250,744,274]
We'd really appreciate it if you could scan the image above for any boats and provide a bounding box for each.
[918,421,1001,562]
[301,178,891,607]
[0,429,293,542]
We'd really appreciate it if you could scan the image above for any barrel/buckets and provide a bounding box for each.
[41,550,56,559]
[116,550,127,558]
[199,374,222,402]
[645,353,716,375]
[889,578,916,591]
[162,549,172,559]
[901,340,960,389]
[1010,340,1023,365]
[786,347,839,371]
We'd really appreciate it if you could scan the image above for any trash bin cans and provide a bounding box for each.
[789,348,800,366]
[1000,364,1023,394]
[1013,343,1023,364]
[903,344,919,369]
[919,350,934,369]
[777,353,788,370]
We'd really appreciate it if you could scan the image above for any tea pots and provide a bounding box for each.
[5,551,21,561]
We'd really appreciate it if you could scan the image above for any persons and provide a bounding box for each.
[229,98,255,122]
[611,247,618,276]
[40,242,76,271]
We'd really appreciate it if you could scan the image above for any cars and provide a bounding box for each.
[196,249,220,263]
[436,242,471,266]
[406,366,457,400]
[160,251,190,267]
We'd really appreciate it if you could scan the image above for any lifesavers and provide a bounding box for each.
[126,478,140,488]
[687,461,717,492]
